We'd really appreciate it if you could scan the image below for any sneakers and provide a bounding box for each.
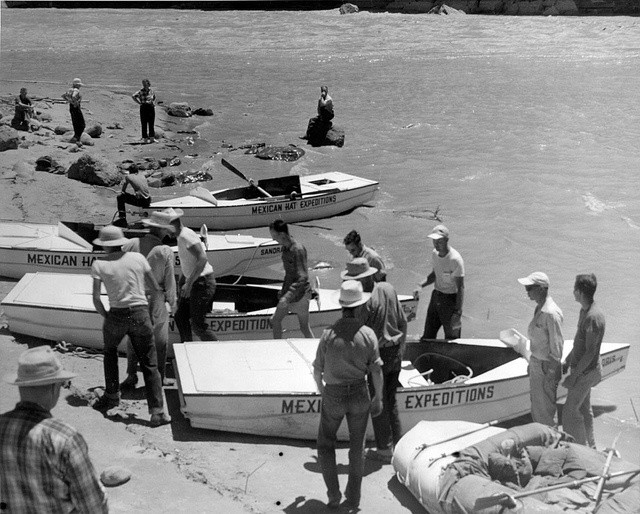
[150,412,171,428]
[365,450,392,464]
[342,499,360,512]
[324,500,340,510]
[112,220,128,226]
[119,374,138,385]
[99,391,121,406]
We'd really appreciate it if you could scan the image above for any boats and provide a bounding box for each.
[173,329,630,441]
[142,172,378,229]
[0,219,284,279]
[0,271,418,354]
[390,420,640,514]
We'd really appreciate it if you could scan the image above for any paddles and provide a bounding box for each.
[473,468,640,511]
[221,159,272,197]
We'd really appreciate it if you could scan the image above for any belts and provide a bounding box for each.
[110,305,148,310]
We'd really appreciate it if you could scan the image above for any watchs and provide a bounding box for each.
[452,309,463,316]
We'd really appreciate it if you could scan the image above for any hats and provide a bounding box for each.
[425,225,449,240]
[140,211,176,233]
[5,344,78,387]
[93,225,131,247]
[517,272,550,288]
[340,258,378,280]
[162,209,184,224]
[331,280,372,308]
[71,78,83,86]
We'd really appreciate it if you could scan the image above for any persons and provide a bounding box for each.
[298,85,333,141]
[62,77,86,143]
[342,257,408,465]
[11,86,36,130]
[114,164,152,229]
[92,226,173,428]
[420,224,464,339]
[315,281,384,511]
[563,274,605,448]
[159,207,217,341]
[519,273,564,426]
[120,211,177,391]
[1,344,107,513]
[344,231,386,281]
[268,218,314,337]
[131,79,155,140]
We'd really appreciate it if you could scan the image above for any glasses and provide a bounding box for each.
[526,285,541,290]
